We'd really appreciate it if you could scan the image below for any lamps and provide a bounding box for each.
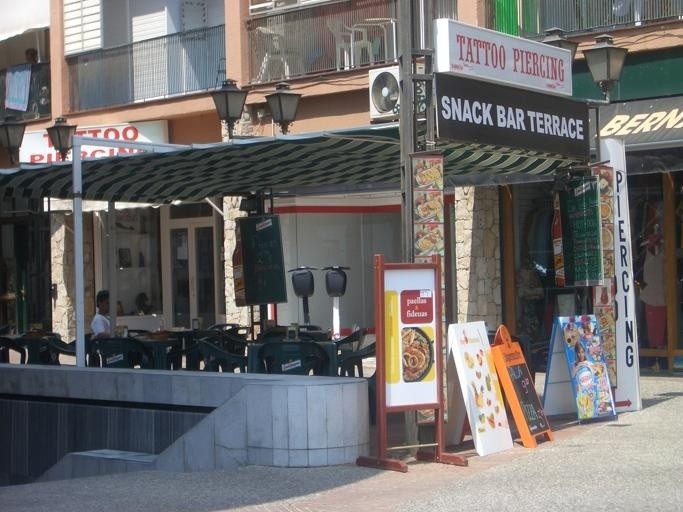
[211,79,302,139]
[0,113,77,164]
[540,25,628,105]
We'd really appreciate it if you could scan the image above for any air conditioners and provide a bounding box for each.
[366,63,424,123]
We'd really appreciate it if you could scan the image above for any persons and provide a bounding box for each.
[25,48,39,65]
[573,341,587,366]
[91,289,110,339]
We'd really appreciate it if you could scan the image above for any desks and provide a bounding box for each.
[350,16,398,68]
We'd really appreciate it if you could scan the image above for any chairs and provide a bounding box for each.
[253,25,305,80]
[1,322,378,378]
[325,17,372,72]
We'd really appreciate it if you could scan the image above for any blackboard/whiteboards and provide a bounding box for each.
[507,363,550,435]
